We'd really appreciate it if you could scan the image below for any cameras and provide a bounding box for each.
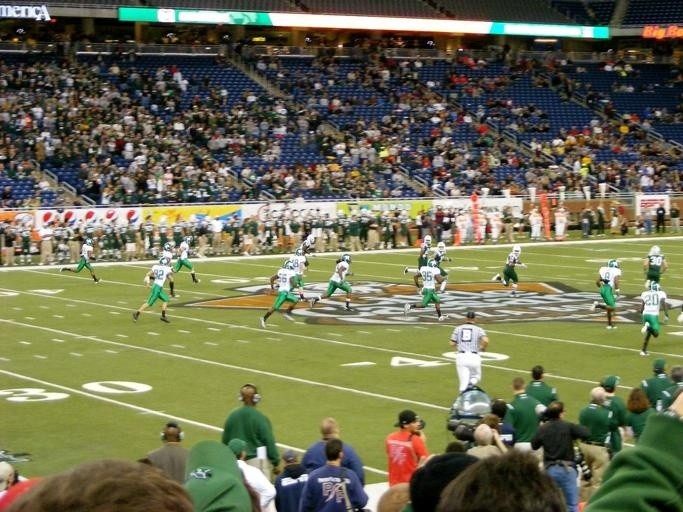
[417,420,425,435]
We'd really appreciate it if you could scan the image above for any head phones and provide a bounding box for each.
[238,383,261,403]
[161,422,184,441]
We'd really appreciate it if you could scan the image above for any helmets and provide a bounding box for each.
[424,235,445,267]
[160,236,192,265]
[513,247,521,254]
[649,280,660,289]
[609,259,617,267]
[343,254,352,262]
[87,239,95,246]
[284,240,310,268]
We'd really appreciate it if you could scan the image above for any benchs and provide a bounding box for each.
[0,56,683,206]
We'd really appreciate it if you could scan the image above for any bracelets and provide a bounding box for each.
[145,276,150,284]
[664,311,668,317]
[513,263,520,267]
[614,288,619,293]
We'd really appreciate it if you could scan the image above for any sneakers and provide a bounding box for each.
[439,314,449,322]
[300,296,317,307]
[344,306,356,312]
[405,304,411,315]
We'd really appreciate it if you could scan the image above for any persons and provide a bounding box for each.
[642,245,667,292]
[220,385,280,473]
[591,259,621,328]
[143,242,180,298]
[0,414,370,511]
[131,257,175,323]
[449,311,488,395]
[637,280,670,356]
[491,245,526,299]
[376,357,683,512]
[0,26,683,262]
[259,234,453,321]
[59,239,101,283]
[168,237,200,285]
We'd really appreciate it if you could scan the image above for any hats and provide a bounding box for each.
[182,439,253,512]
[601,376,620,387]
[394,411,417,426]
